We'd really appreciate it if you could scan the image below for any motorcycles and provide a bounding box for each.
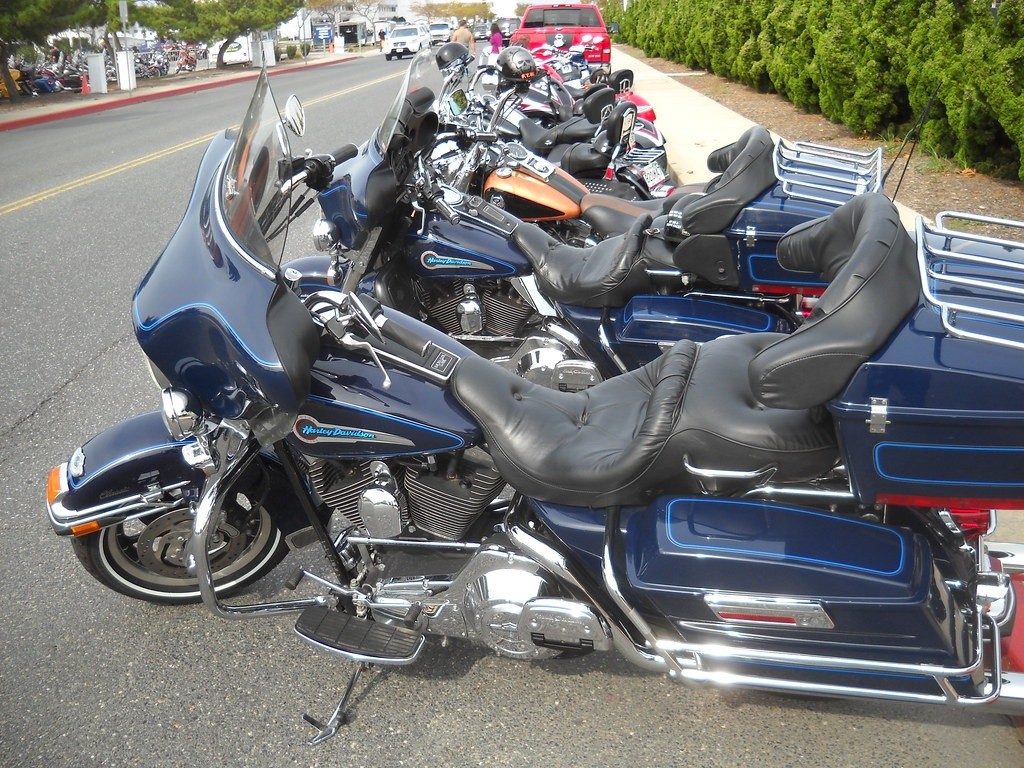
[43,39,1022,746]
[18,40,197,97]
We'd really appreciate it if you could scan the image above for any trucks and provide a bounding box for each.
[373,19,396,46]
[337,20,367,47]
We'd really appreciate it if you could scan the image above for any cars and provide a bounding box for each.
[473,25,491,43]
[509,5,618,68]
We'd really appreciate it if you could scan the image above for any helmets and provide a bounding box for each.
[497,45,537,82]
[436,42,469,70]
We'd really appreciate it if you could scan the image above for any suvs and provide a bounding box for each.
[495,18,521,43]
[382,24,432,62]
[429,22,452,45]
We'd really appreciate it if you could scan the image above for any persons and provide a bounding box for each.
[50,46,60,63]
[451,20,476,78]
[378,29,386,52]
[490,23,503,55]
[133,46,139,53]
